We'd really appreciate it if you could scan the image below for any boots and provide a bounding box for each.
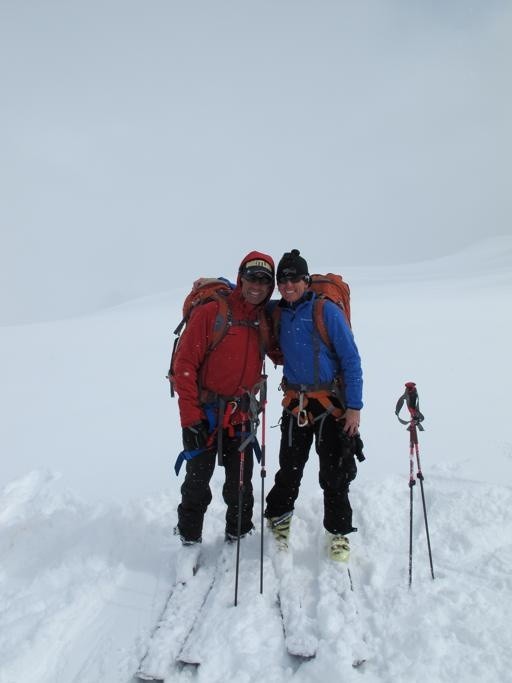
[267,511,295,572]
[328,534,352,564]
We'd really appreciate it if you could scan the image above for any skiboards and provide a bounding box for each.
[271,549,367,665]
[132,535,229,681]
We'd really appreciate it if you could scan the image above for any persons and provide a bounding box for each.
[170,252,286,561]
[262,249,365,560]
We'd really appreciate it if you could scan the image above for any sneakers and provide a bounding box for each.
[177,536,204,586]
[218,532,245,572]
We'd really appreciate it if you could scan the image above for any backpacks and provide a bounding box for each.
[271,272,354,354]
[167,276,269,394]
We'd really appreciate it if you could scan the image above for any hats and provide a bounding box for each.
[275,248,310,276]
[243,258,274,278]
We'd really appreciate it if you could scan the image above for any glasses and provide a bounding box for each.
[277,276,309,282]
[241,273,273,285]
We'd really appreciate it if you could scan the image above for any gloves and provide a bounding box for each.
[182,419,209,452]
